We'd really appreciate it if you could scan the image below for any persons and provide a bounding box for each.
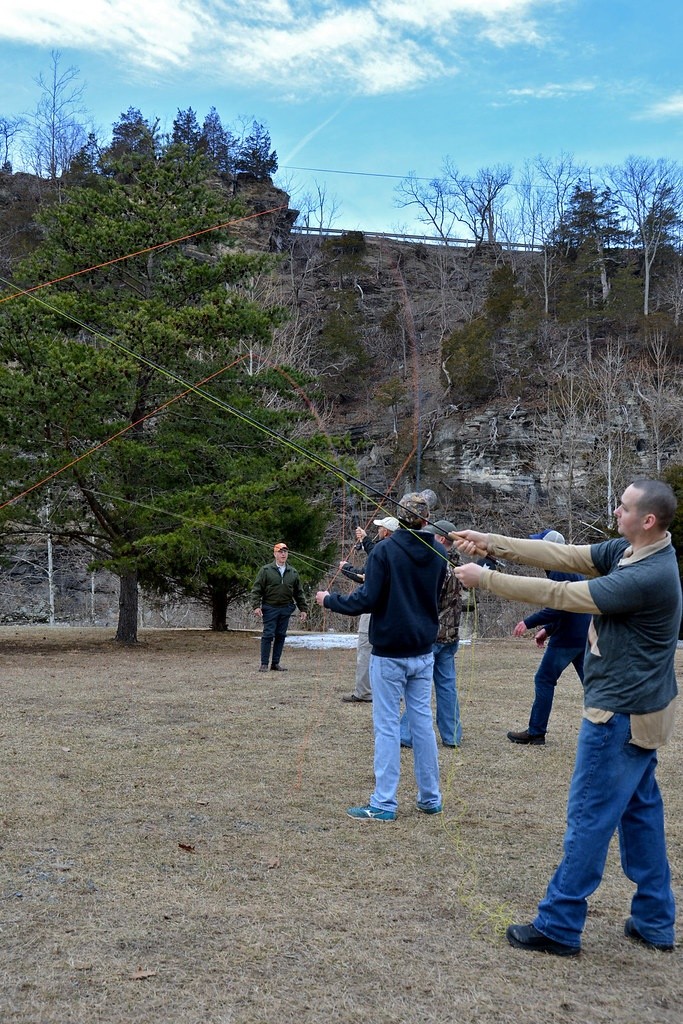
[316,492,463,822]
[507,528,592,745]
[249,543,308,671]
[451,478,683,957]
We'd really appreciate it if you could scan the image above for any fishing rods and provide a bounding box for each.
[82,486,367,584]
[1,276,515,598]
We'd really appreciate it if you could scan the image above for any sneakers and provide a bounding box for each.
[415,804,443,815]
[342,694,372,702]
[347,803,397,822]
[507,730,546,745]
[624,917,676,953]
[506,923,581,957]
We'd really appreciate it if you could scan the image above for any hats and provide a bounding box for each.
[373,517,400,532]
[529,529,566,545]
[274,543,289,552]
[423,520,457,541]
[397,489,438,527]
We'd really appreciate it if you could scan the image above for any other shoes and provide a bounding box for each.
[270,664,288,671]
[259,664,268,672]
